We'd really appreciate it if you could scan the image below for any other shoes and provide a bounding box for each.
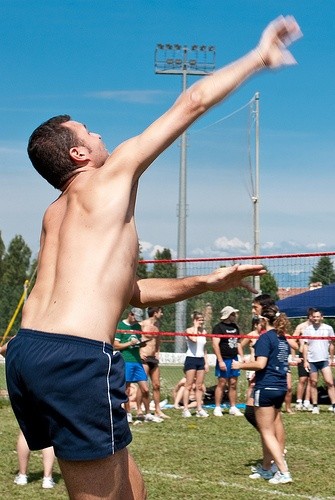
[13,474,28,485]
[328,406,335,413]
[229,406,244,417]
[269,461,289,472]
[303,404,313,411]
[296,401,303,410]
[248,469,273,480]
[268,471,293,484]
[195,409,210,417]
[126,413,133,422]
[42,475,56,489]
[144,414,165,423]
[213,406,224,416]
[136,411,143,417]
[312,407,319,415]
[182,409,192,418]
[154,411,172,419]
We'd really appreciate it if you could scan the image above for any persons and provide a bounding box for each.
[0,334,55,488]
[0,15,303,500]
[139,305,171,423]
[173,367,206,408]
[182,311,209,418]
[244,295,288,479]
[114,307,163,424]
[241,307,335,415]
[231,305,293,483]
[212,306,244,418]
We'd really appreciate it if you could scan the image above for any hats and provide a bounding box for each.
[131,307,144,322]
[219,305,239,320]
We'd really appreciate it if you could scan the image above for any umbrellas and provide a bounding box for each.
[273,284,335,317]
